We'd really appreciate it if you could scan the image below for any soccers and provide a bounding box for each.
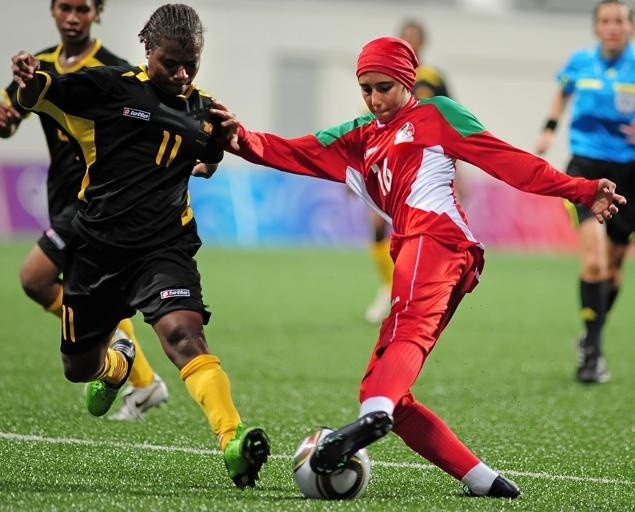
[291,428,370,500]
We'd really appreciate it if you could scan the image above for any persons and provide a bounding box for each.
[536,0,635,384]
[10,3,272,491]
[0,0,173,424]
[208,36,628,500]
[364,20,450,325]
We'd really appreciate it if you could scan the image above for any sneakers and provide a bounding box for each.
[109,371,168,423]
[308,410,394,477]
[223,422,272,490]
[85,339,136,416]
[463,474,522,499]
[576,336,609,384]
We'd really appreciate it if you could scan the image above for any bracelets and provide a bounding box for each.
[543,118,558,131]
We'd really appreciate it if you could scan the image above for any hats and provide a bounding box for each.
[356,37,420,93]
